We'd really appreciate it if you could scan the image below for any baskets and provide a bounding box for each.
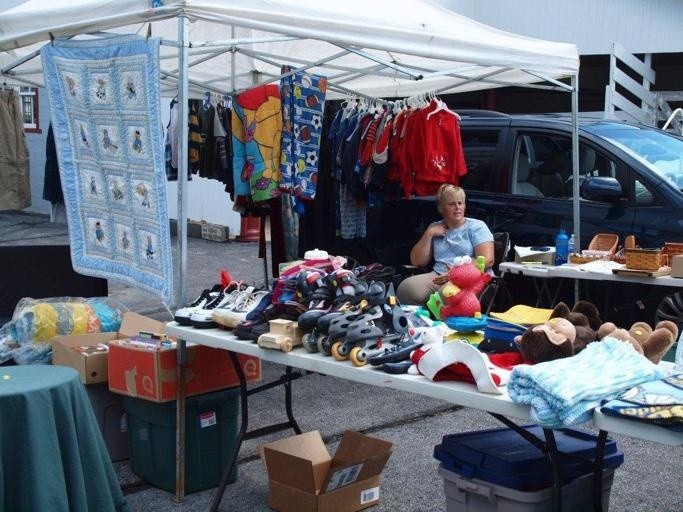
[570,234,683,269]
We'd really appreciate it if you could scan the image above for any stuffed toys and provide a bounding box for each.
[596,320,679,365]
[423,255,492,321]
[512,300,604,366]
[406,325,447,376]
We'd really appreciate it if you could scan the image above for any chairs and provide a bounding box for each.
[402,232,515,315]
[517,143,596,198]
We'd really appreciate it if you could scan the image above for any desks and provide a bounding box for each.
[0,364,131,512]
[167,321,607,512]
[498,262,683,309]
[593,406,683,447]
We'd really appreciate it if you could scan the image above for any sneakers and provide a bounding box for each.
[174,276,300,338]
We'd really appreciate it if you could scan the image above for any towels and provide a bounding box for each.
[507,336,663,430]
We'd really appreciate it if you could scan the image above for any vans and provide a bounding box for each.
[295,106,683,346]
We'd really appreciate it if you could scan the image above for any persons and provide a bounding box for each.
[395,182,498,306]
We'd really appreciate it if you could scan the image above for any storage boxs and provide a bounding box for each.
[434,424,624,512]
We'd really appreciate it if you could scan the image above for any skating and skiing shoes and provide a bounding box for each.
[298,263,425,374]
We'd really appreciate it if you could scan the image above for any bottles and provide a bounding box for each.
[567,233,576,253]
[555,220,568,265]
[475,255,485,272]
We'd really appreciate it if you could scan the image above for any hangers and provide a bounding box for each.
[341,89,462,121]
[199,92,230,109]
[174,85,179,101]
